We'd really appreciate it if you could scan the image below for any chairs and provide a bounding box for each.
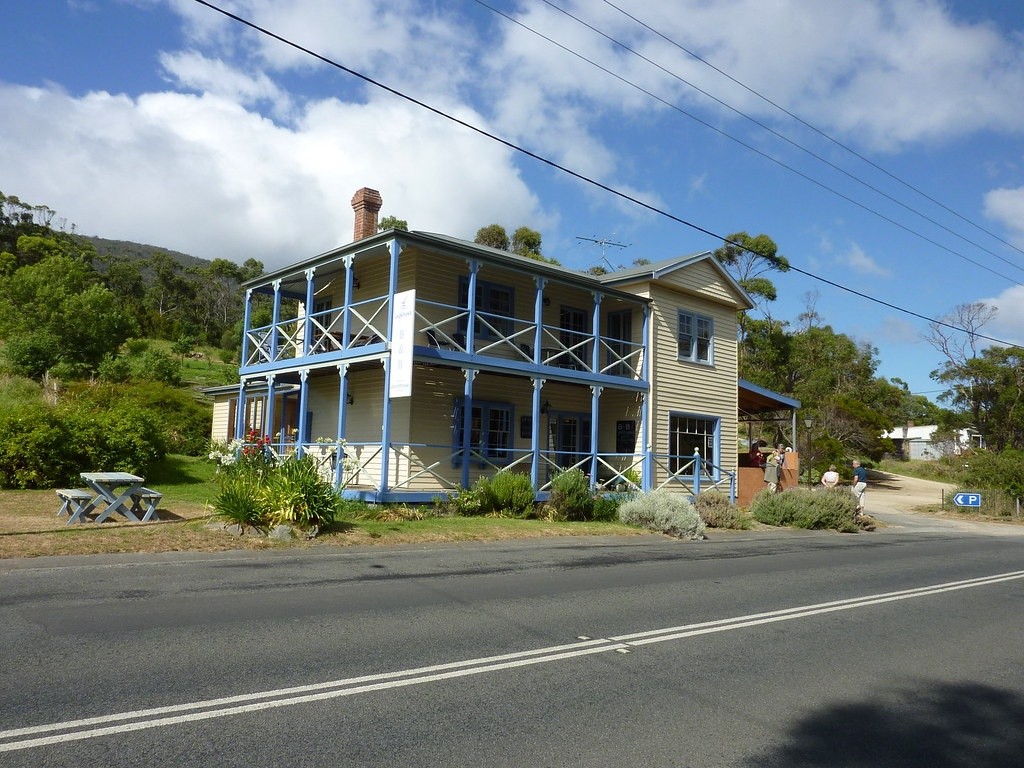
[330,453,353,486]
[521,344,534,360]
[453,334,466,352]
[427,330,449,349]
[557,353,576,371]
[258,332,286,364]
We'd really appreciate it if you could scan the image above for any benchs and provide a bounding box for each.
[55,489,93,526]
[130,487,163,522]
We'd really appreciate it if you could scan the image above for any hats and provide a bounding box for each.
[777,443,786,449]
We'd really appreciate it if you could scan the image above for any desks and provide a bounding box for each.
[541,346,563,366]
[80,472,144,523]
[314,332,369,352]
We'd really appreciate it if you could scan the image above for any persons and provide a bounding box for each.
[820,464,840,488]
[850,459,868,517]
[748,440,793,495]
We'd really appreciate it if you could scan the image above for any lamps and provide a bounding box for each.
[353,273,360,290]
[540,400,552,414]
[347,393,353,405]
[542,298,551,306]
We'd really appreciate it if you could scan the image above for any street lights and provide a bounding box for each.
[803,413,815,492]
